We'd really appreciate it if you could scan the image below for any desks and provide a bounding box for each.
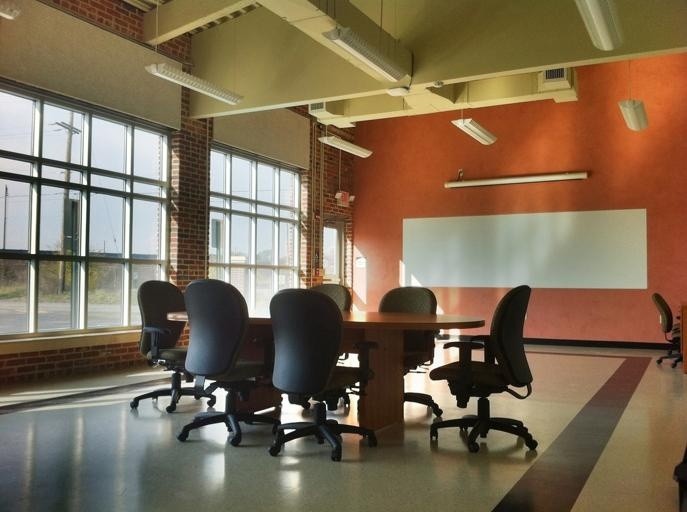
[163,306,484,439]
[678,299,687,373]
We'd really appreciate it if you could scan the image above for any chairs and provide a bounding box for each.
[130,278,217,412]
[178,278,284,448]
[299,283,352,410]
[354,285,442,424]
[427,283,538,456]
[265,288,378,461]
[650,292,685,368]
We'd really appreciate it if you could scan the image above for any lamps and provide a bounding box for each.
[449,81,497,146]
[615,60,650,131]
[143,0,245,106]
[321,1,408,84]
[575,1,626,51]
[317,124,373,159]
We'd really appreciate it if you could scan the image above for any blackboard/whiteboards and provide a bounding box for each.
[402,207,649,290]
